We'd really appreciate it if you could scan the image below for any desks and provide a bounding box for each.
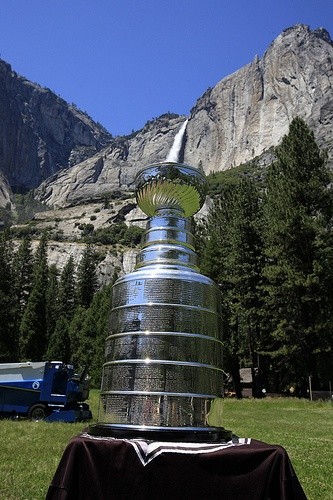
[45,435,307,500]
